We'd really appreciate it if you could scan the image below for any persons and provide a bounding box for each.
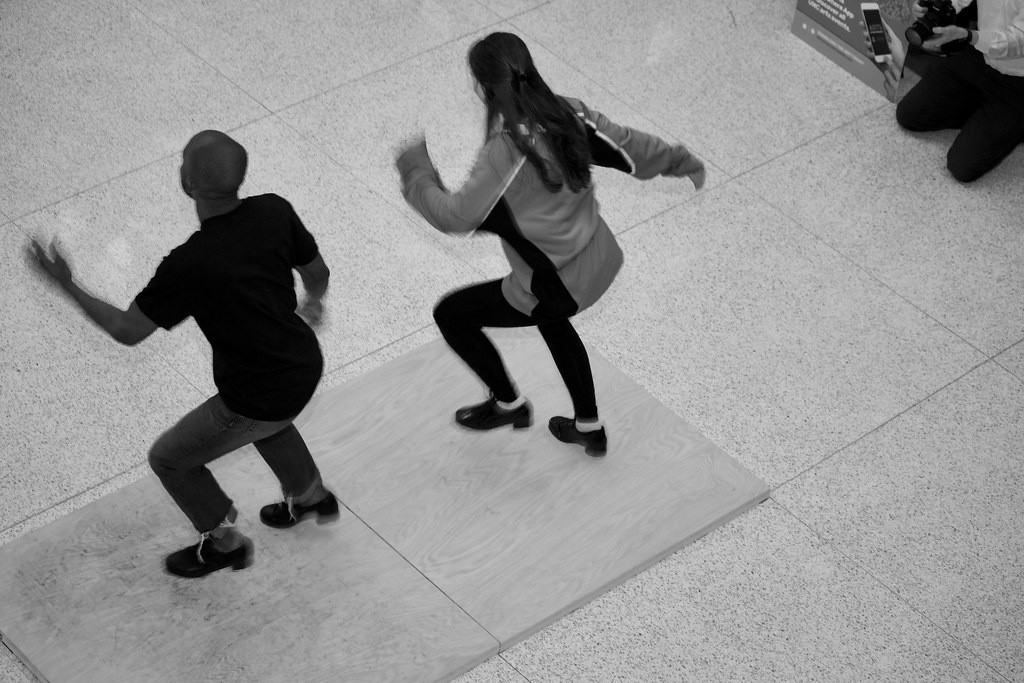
[396,33,706,457]
[896,0,1024,183]
[858,16,905,103]
[24,130,339,578]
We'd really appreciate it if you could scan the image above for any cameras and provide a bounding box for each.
[905,0,956,47]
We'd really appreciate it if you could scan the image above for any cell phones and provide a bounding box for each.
[860,3,893,63]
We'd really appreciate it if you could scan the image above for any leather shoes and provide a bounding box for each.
[455,396,530,430]
[548,416,607,457]
[166,538,249,578]
[260,490,338,529]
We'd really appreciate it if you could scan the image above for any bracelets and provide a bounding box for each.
[965,29,973,45]
